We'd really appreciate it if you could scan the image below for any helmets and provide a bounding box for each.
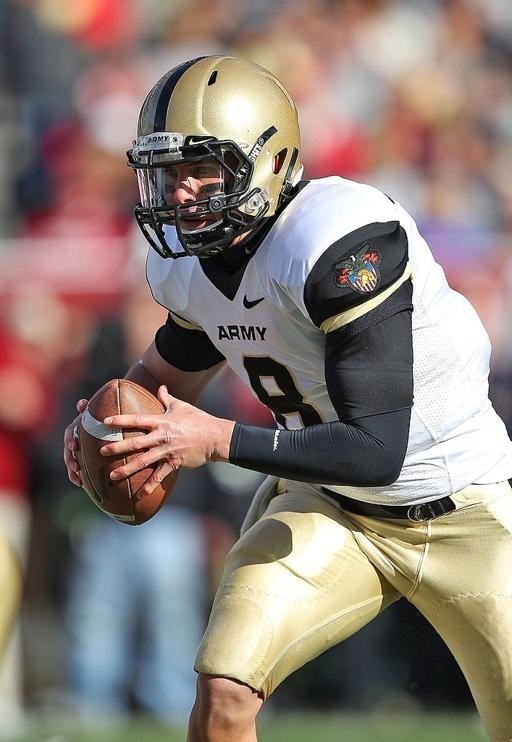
[126,54,301,258]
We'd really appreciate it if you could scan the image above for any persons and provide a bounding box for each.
[62,50,512,742]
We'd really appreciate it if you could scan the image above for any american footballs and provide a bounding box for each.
[73,379,179,526]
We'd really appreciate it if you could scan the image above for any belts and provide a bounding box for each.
[323,488,456,519]
